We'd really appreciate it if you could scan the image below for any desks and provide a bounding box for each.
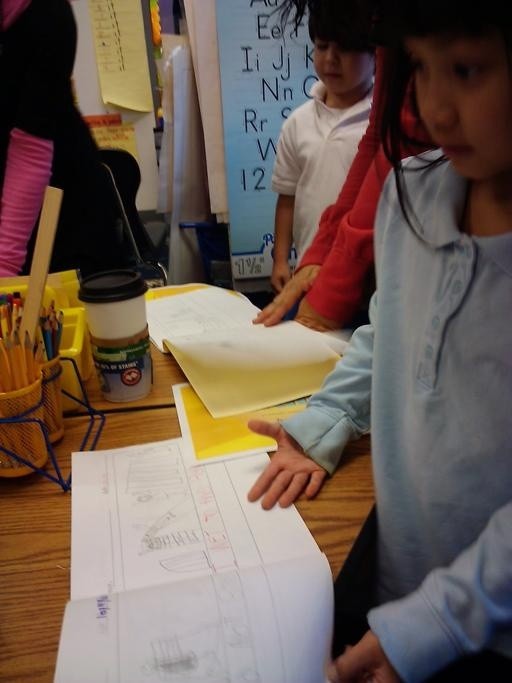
[0,335,375,682]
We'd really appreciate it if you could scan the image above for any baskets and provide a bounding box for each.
[0,371,48,479]
[40,354,64,444]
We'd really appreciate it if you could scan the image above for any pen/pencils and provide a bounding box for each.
[0,292,62,469]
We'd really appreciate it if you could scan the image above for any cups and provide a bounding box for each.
[77,271,153,404]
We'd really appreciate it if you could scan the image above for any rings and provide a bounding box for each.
[272,298,281,305]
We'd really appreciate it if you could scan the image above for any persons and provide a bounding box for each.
[0,0,120,281]
[253,2,439,332]
[271,0,375,320]
[249,0,510,683]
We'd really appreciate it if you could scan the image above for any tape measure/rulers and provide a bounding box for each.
[18,186,63,361]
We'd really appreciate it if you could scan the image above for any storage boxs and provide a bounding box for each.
[0,268,97,414]
